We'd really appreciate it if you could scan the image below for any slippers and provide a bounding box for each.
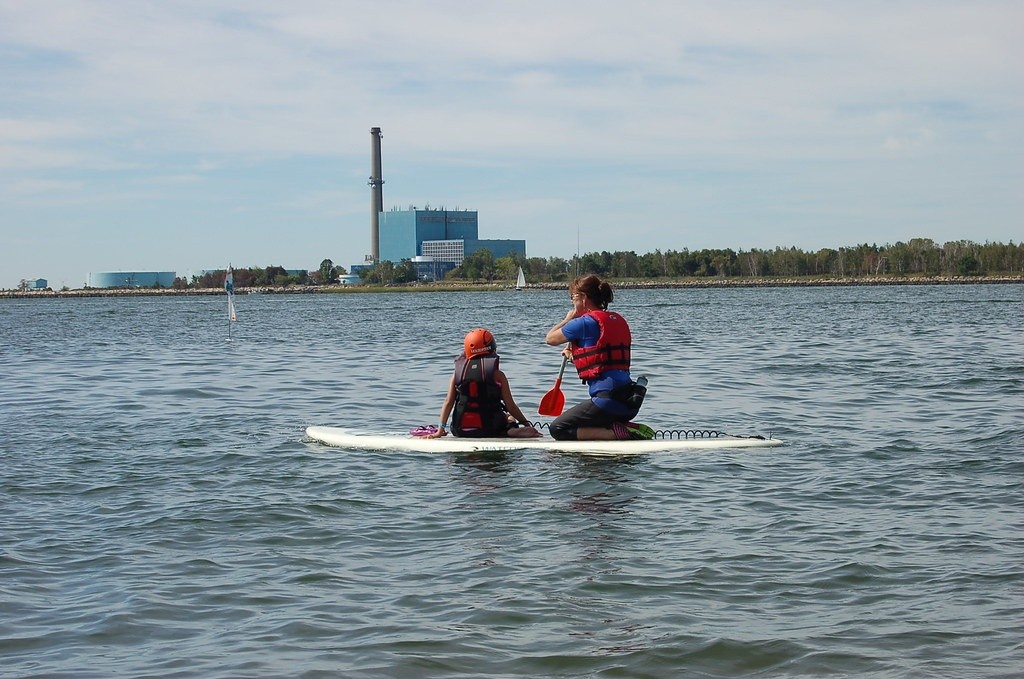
[425,425,448,436]
[410,426,435,435]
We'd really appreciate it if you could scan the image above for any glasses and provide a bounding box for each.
[570,293,585,301]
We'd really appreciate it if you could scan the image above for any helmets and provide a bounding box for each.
[464,330,497,360]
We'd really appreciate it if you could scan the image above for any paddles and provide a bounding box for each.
[537,342,572,417]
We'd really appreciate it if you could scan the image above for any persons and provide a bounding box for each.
[425,328,539,438]
[545,275,656,441]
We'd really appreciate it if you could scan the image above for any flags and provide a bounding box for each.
[225,263,239,322]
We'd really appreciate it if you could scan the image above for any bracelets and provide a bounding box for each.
[439,422,446,428]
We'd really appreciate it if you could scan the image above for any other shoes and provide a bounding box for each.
[610,423,647,439]
[625,421,654,438]
